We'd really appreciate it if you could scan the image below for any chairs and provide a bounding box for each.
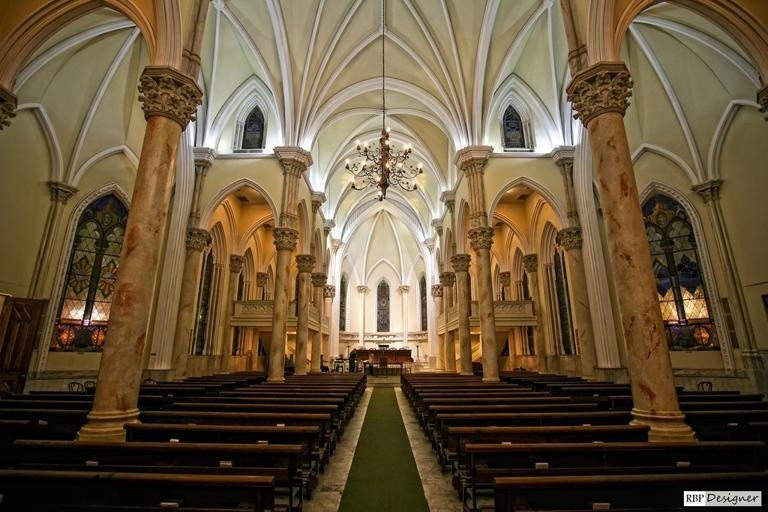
[405,358,413,376]
[379,357,387,376]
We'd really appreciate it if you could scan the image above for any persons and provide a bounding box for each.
[320,355,329,371]
[367,350,374,374]
[335,355,343,372]
[347,347,357,372]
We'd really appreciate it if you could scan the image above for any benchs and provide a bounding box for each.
[479,471,768,512]
[447,424,651,490]
[457,439,768,512]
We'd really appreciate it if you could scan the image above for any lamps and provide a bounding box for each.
[344,0,423,203]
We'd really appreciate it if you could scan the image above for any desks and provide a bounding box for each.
[355,349,414,375]
[334,360,344,373]
